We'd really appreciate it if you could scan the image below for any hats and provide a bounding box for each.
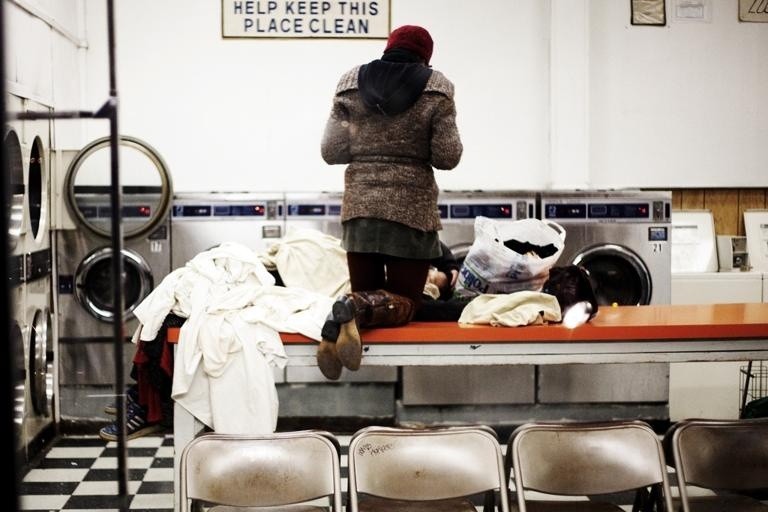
[385,24,433,62]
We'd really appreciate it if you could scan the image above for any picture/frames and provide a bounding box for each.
[738,1,767,24]
[630,0,666,26]
[220,1,392,39]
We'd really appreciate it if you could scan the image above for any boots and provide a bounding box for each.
[316,289,415,380]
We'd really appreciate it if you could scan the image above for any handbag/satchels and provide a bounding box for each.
[455,215,567,296]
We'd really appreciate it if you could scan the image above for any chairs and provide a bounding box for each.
[181,419,767,511]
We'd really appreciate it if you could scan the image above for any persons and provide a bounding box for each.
[423,241,460,301]
[317,26,463,380]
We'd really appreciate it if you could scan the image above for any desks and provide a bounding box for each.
[167,303,768,512]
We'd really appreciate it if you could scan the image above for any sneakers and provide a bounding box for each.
[99,392,166,441]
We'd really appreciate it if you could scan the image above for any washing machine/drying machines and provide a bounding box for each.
[400,192,540,407]
[48,132,173,429]
[285,198,399,420]
[5,92,56,458]
[543,189,674,404]
[169,191,288,264]
